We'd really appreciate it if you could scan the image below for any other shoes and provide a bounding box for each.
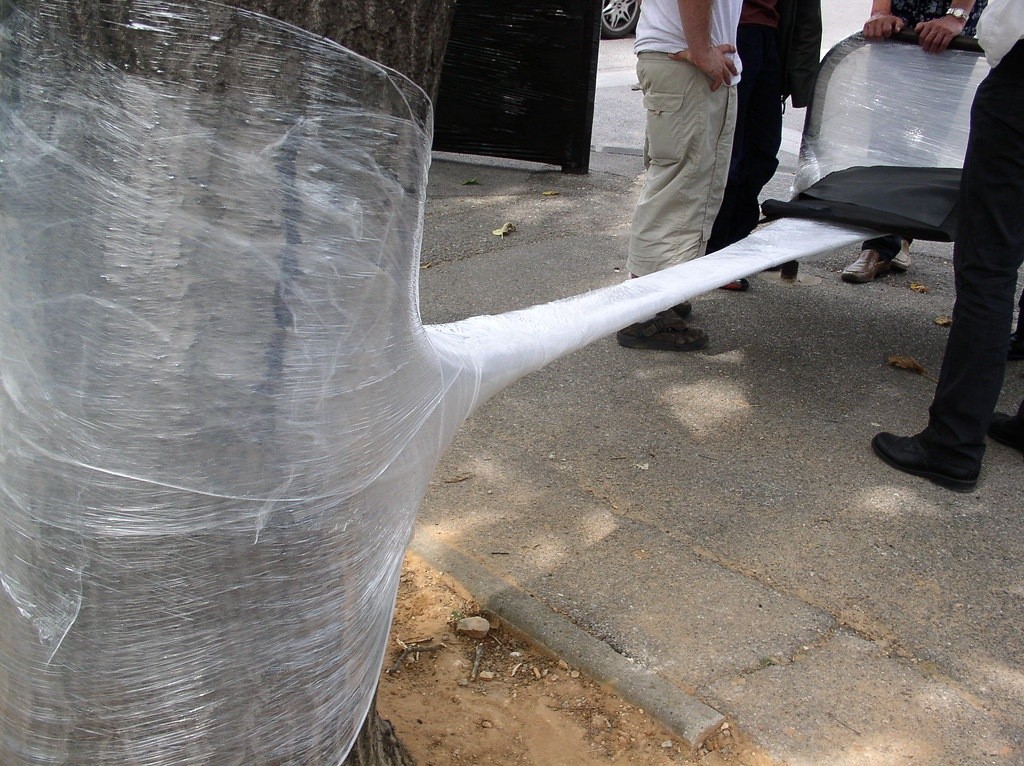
[721,276,749,291]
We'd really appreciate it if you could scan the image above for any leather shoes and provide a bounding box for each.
[871,431,980,493]
[840,249,891,284]
[890,239,911,270]
[986,411,1024,456]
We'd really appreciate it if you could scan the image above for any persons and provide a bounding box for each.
[843,0,985,285]
[871,0,1024,493]
[706,0,822,290]
[618,0,743,353]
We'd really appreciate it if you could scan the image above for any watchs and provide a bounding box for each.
[946,8,969,22]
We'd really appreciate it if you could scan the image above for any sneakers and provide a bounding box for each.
[617,309,710,351]
[671,300,692,316]
